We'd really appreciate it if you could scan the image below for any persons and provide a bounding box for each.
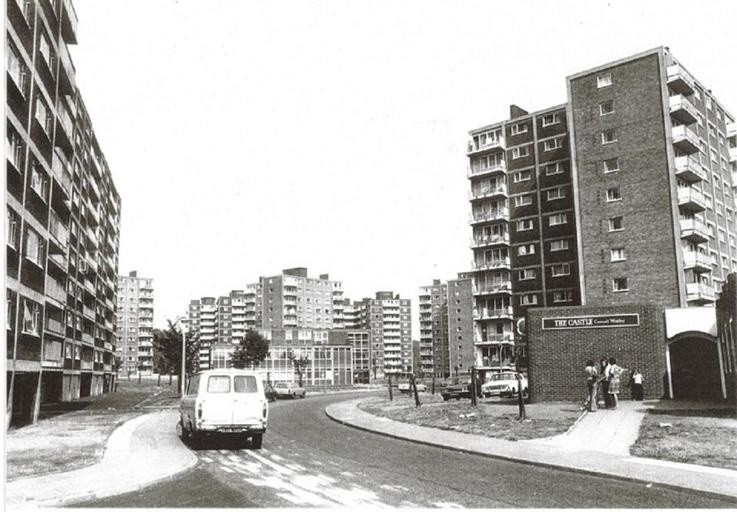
[632,369,646,401]
[585,357,622,411]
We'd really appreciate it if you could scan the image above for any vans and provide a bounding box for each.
[180,368,269,449]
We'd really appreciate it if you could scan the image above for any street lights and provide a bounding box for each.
[178,319,194,395]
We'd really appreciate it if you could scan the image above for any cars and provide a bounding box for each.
[273,382,306,399]
[439,366,528,401]
[398,379,427,393]
